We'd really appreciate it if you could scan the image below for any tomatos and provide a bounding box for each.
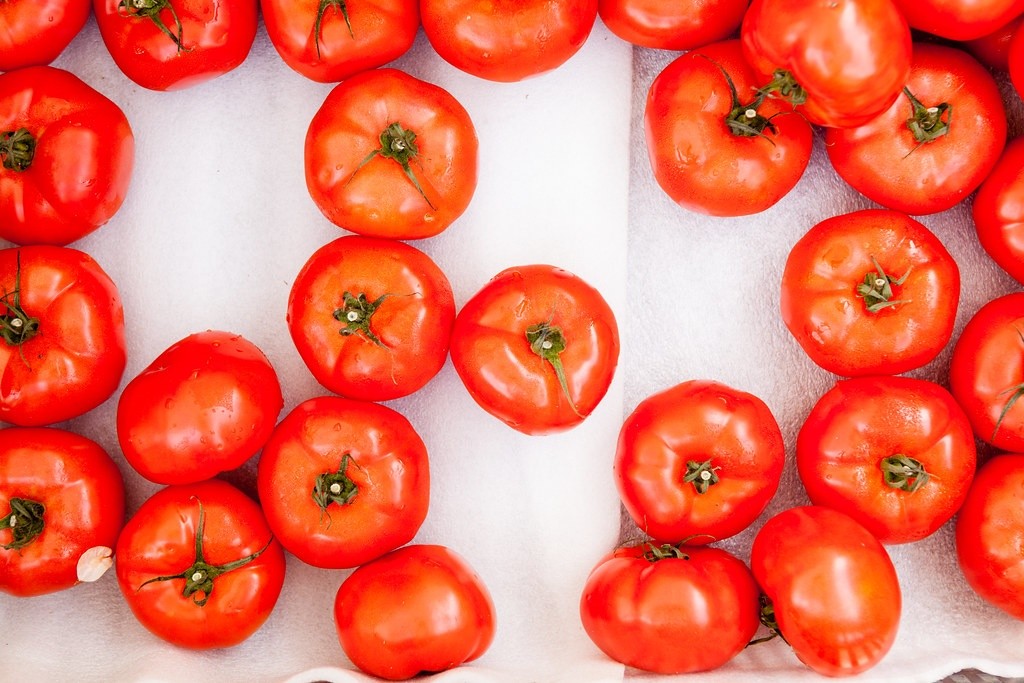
[0,0,1024,682]
